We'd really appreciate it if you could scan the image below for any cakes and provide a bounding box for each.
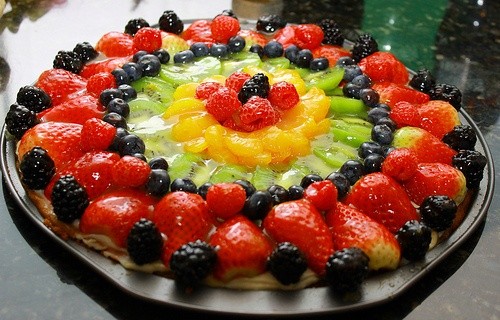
[3,8,487,291]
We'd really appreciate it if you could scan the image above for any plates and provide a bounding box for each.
[0,18,493,315]
